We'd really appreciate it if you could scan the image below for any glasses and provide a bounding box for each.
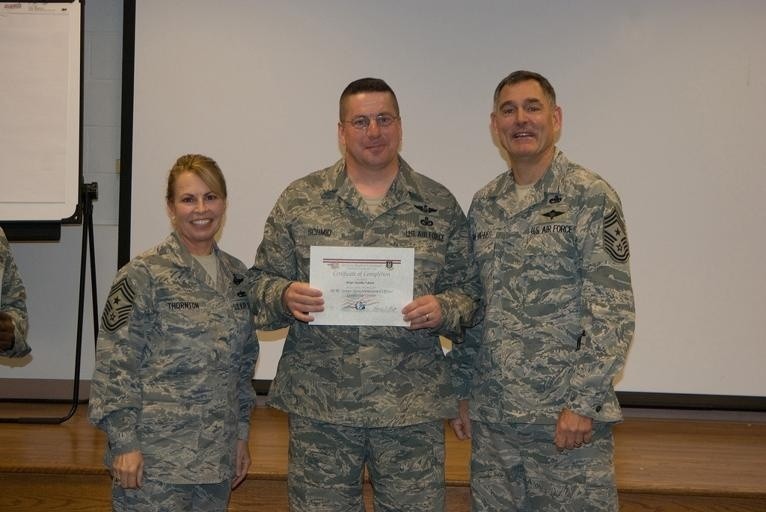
[344,112,399,130]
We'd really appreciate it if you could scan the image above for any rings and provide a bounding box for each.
[575,441,583,445]
[115,477,121,482]
[426,314,429,321]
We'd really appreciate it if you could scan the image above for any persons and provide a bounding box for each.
[87,155,260,512]
[0,225,32,359]
[246,77,486,512]
[446,71,635,512]
